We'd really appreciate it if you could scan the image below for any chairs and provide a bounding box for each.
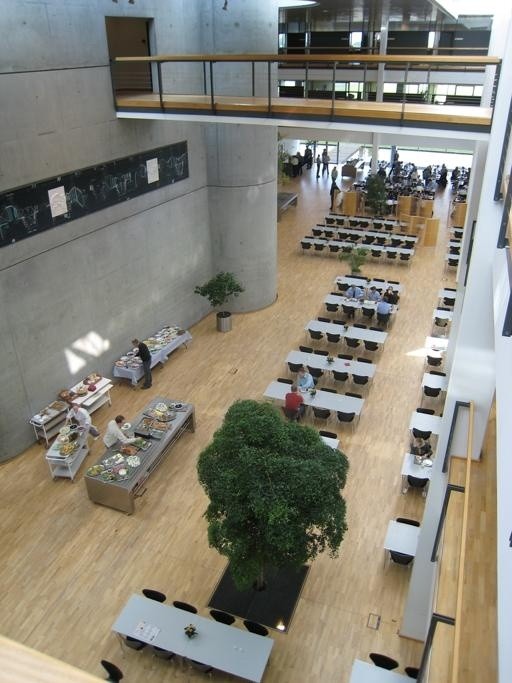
[383,514,420,575]
[98,657,128,683]
[398,287,457,497]
[125,587,271,680]
[446,225,465,271]
[301,211,418,265]
[263,273,402,449]
[369,651,420,680]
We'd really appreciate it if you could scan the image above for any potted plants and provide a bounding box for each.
[193,271,246,333]
[338,247,373,279]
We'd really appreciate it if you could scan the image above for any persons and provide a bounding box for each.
[367,287,381,318]
[64,404,101,449]
[132,339,152,389]
[103,415,142,450]
[297,367,315,391]
[345,284,363,319]
[291,146,471,209]
[410,438,432,459]
[377,296,391,323]
[286,385,304,421]
[383,287,399,304]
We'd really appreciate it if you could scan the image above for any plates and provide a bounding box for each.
[87,452,140,484]
[31,401,67,424]
[59,372,102,400]
[133,416,171,441]
[118,436,152,456]
[116,325,186,371]
[142,402,184,421]
[422,458,432,468]
[120,423,132,430]
[52,424,87,456]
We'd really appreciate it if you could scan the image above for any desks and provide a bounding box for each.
[114,324,194,392]
[348,659,415,683]
[111,595,277,682]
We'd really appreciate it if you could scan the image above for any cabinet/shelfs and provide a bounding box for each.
[30,375,200,515]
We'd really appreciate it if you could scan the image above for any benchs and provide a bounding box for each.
[278,191,298,219]
